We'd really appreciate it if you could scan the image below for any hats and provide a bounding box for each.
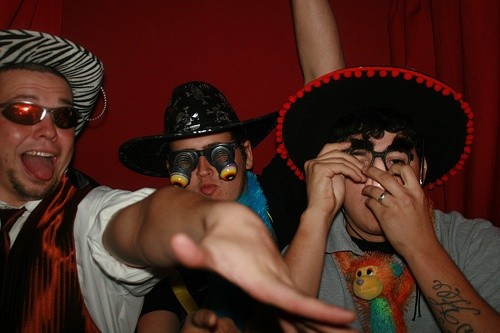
[118,81,280,176]
[0,29,107,136]
[276,68,474,193]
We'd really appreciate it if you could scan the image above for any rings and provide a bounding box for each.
[378,192,387,203]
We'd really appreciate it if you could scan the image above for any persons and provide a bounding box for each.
[274,65,500,333]
[0,28,359,333]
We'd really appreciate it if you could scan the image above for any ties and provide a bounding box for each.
[0,206,27,305]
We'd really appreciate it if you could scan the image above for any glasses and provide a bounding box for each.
[0,102,80,129]
[347,135,415,176]
[164,141,240,187]
